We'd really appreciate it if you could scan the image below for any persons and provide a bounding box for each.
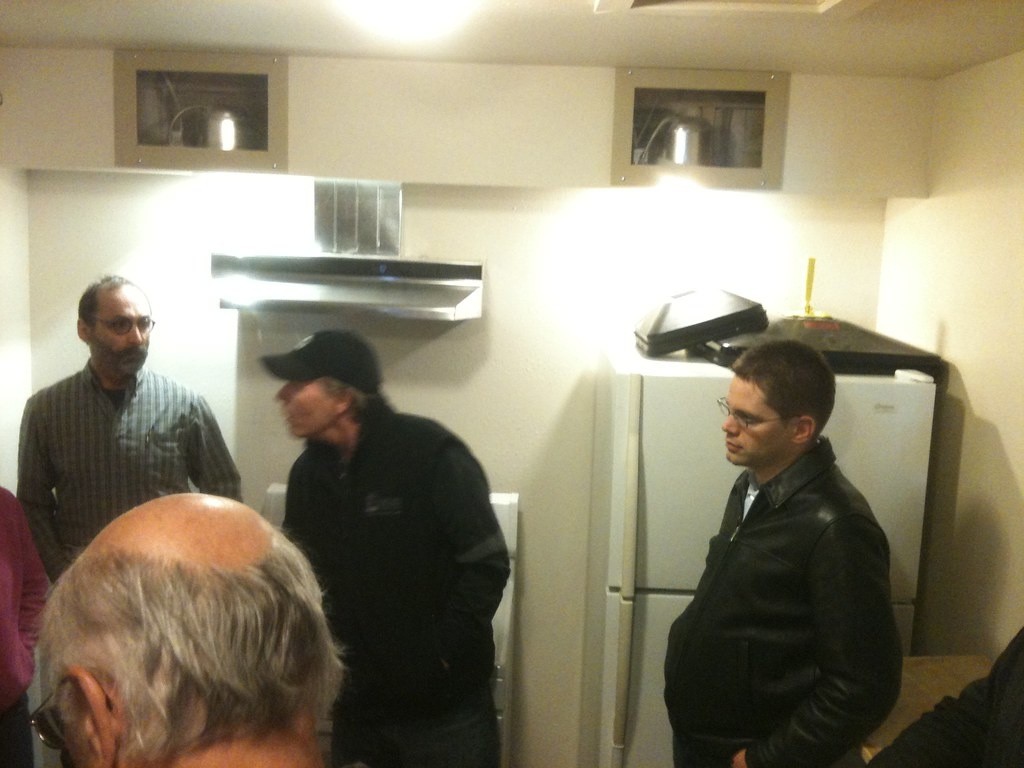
[662,339,1024,767]
[17,278,243,581]
[0,486,52,768]
[260,332,512,768]
[31,492,348,768]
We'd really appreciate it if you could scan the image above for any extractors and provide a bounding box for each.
[211,250,483,321]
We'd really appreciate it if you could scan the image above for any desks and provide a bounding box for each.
[858,653,996,764]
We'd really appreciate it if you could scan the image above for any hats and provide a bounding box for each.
[258,330,381,396]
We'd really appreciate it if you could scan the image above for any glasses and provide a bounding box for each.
[90,315,156,336]
[27,669,114,750]
[716,395,801,429]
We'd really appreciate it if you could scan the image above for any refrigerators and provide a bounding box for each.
[577,337,938,768]
